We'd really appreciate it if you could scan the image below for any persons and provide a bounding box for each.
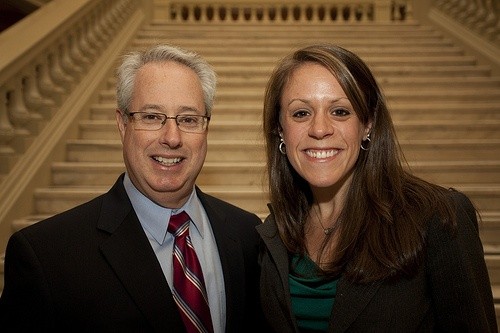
[253,41,499,333]
[0,43,271,333]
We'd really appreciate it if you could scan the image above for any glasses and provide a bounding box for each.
[125,110,210,134]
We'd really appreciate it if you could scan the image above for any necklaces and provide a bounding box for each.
[312,203,342,235]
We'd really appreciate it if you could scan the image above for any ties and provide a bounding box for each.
[167,211,214,333]
